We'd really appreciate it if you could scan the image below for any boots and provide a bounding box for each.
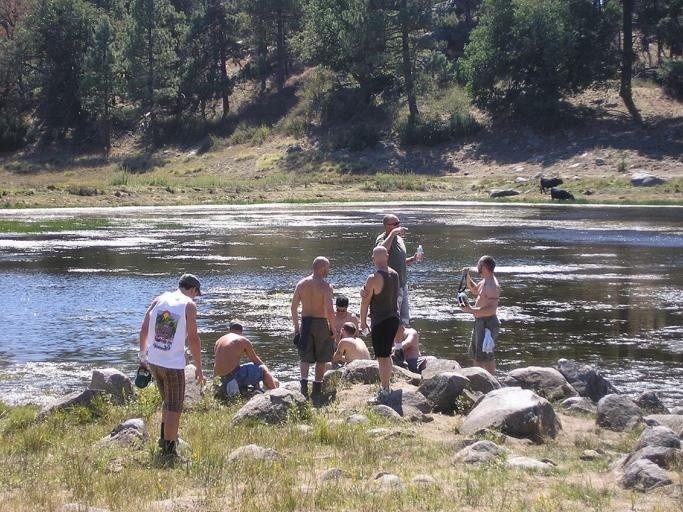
[310,380,330,399]
[300,379,310,400]
[160,439,177,457]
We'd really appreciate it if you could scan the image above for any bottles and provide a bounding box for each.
[416,244,423,266]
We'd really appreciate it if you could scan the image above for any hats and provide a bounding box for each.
[179,273,201,297]
[336,297,348,305]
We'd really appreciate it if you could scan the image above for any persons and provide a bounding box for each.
[291,214,419,407]
[138,273,204,469]
[458,256,500,377]
[213,323,278,394]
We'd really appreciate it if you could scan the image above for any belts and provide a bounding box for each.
[222,366,240,380]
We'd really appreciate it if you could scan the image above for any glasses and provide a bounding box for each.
[337,308,346,312]
[387,221,401,226]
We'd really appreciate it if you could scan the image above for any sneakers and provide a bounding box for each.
[366,385,393,406]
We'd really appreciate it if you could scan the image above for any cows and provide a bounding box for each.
[550,186,575,203]
[540,176,563,195]
